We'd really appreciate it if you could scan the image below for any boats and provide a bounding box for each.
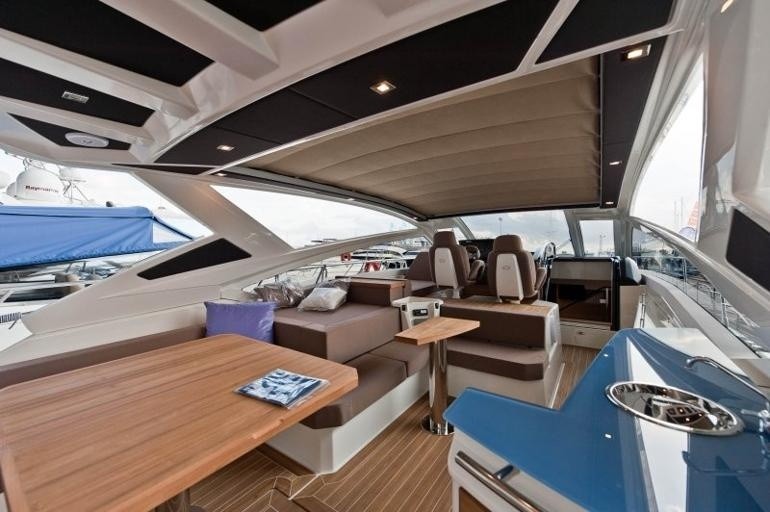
[0,148,199,304]
[293,228,429,267]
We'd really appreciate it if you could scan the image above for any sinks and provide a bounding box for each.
[604,381,746,438]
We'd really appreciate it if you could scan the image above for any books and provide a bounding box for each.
[236,370,329,412]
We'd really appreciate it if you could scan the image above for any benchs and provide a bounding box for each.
[264,275,431,473]
[0,325,207,384]
[432,273,566,409]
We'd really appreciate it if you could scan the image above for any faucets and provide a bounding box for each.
[680,353,766,399]
[683,447,766,477]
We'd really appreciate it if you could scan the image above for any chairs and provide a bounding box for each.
[429,230,485,294]
[486,235,549,304]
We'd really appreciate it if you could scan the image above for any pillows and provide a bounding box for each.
[254,282,298,311]
[203,300,276,345]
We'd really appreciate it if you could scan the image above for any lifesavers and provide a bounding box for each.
[364,260,380,271]
[341,252,350,263]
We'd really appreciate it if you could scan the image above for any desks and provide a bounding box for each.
[0,333,360,512]
